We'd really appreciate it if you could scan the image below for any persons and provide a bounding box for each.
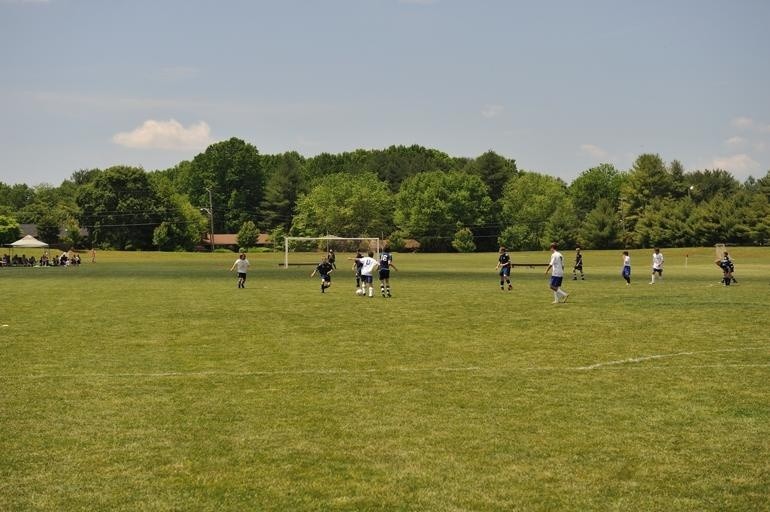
[714,259,734,286]
[328,249,336,269]
[347,245,399,298]
[230,252,250,289]
[311,256,333,293]
[719,251,738,284]
[495,246,513,293]
[544,242,569,304]
[0,247,97,267]
[649,247,664,285]
[572,248,584,280]
[620,250,631,287]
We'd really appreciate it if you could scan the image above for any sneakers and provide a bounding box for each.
[368,294,374,298]
[237,283,246,289]
[382,294,385,298]
[500,286,514,290]
[387,293,392,297]
[625,282,655,287]
[563,295,570,303]
[362,292,367,296]
[572,277,585,281]
[320,286,329,292]
[552,301,560,304]
[720,276,738,286]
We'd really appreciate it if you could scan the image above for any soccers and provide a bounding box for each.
[356,289,363,295]
